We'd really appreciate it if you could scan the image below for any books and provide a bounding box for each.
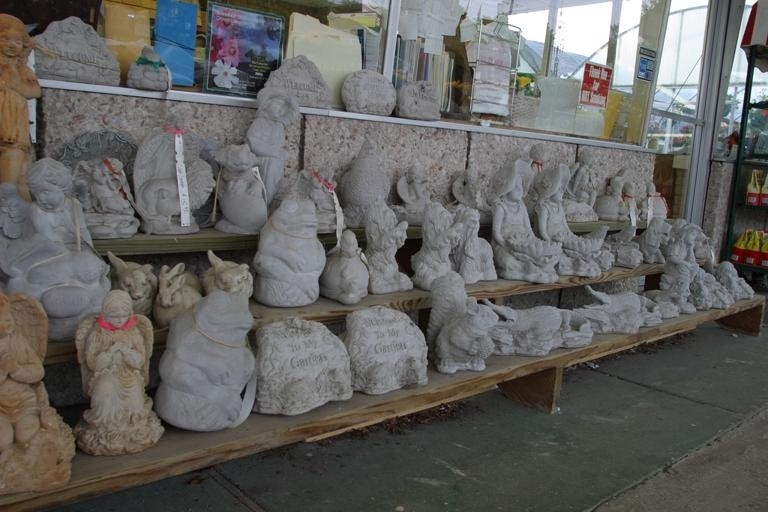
[397,32,457,112]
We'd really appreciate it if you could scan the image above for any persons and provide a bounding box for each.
[659,223,703,312]
[84,291,151,427]
[13,157,105,286]
[490,159,562,273]
[534,163,615,276]
[0,13,41,183]
[248,87,295,205]
[0,290,44,451]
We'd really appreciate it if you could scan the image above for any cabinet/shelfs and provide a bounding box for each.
[0,219,765,512]
[440,16,521,128]
[721,0,768,272]
